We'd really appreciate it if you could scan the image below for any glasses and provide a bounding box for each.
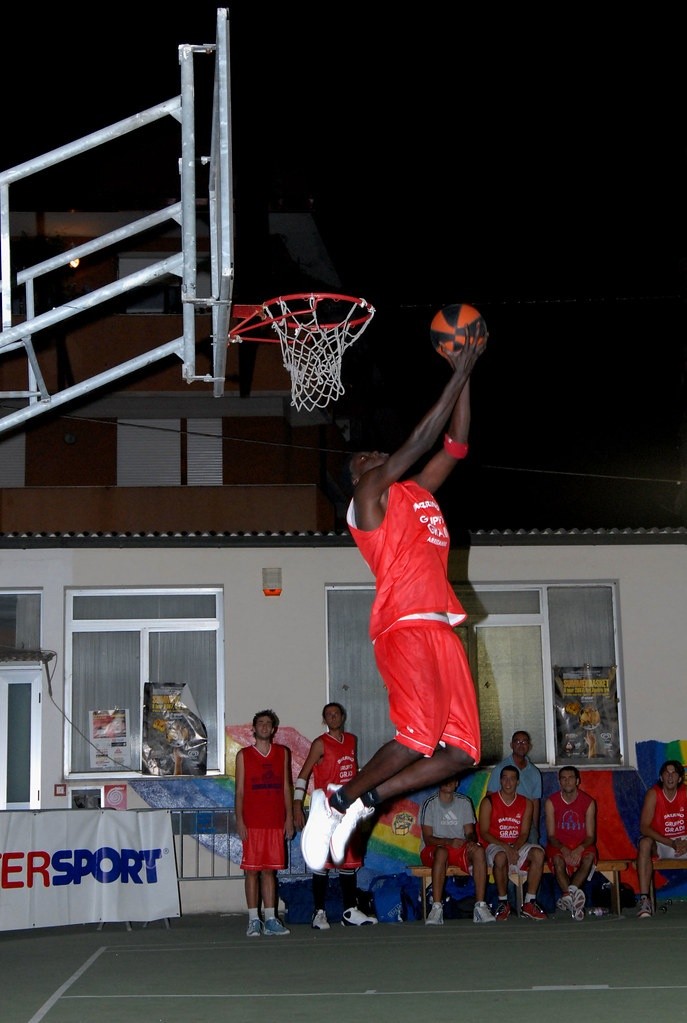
[514,740,528,745]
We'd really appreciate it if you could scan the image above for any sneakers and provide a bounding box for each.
[637,895,652,918]
[311,907,331,929]
[556,889,585,921]
[246,918,264,937]
[494,900,511,921]
[326,783,376,865]
[300,787,347,870]
[425,901,444,926]
[522,899,547,920]
[263,918,291,935]
[340,905,378,926]
[473,900,497,923]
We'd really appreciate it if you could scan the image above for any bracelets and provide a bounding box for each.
[443,433,469,459]
[477,842,482,847]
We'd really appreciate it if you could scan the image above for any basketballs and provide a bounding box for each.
[429,303,488,360]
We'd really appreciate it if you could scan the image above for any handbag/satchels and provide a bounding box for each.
[368,873,421,923]
[590,882,637,908]
[277,877,348,924]
[457,894,478,919]
[419,882,458,920]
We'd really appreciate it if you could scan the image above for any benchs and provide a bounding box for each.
[634,859,687,914]
[410,860,627,921]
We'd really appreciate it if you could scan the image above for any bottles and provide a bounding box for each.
[588,908,609,915]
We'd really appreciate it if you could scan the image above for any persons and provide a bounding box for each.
[479,766,548,921]
[488,731,543,845]
[544,766,596,921]
[419,781,495,926]
[294,703,379,929]
[637,760,687,919]
[234,710,295,937]
[301,322,491,869]
